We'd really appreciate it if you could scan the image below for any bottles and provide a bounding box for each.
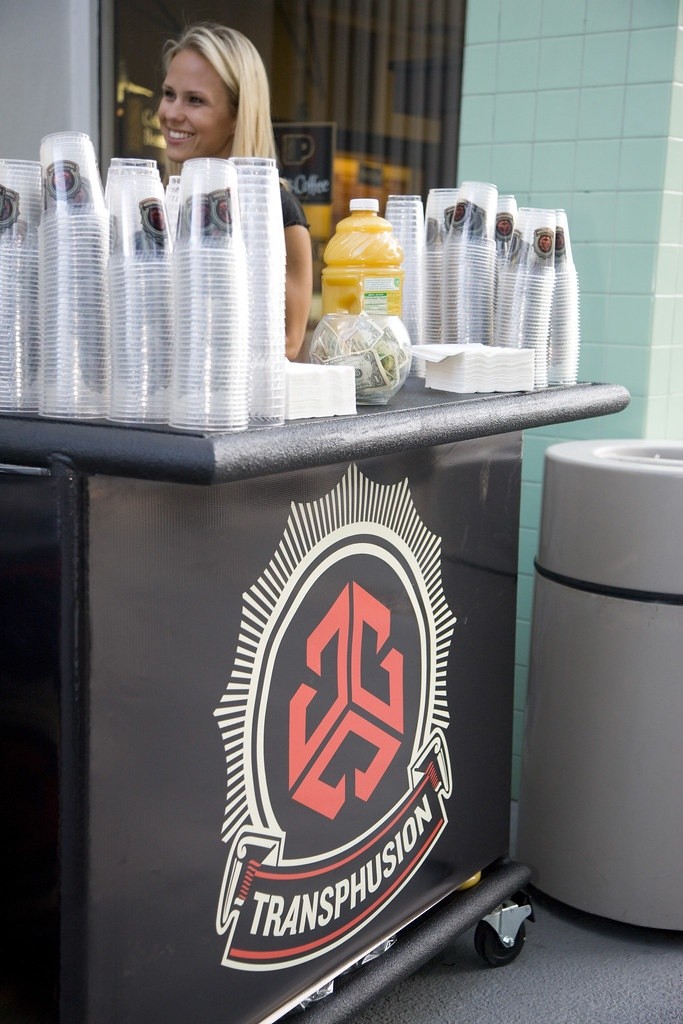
[320,197,407,340]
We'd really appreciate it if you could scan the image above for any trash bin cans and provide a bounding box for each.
[517,436,682,936]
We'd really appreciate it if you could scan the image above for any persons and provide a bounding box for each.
[158,22,313,361]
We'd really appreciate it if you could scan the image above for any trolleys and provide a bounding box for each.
[1,375,632,1024]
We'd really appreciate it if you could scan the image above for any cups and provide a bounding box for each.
[1,133,287,434]
[389,184,581,391]
